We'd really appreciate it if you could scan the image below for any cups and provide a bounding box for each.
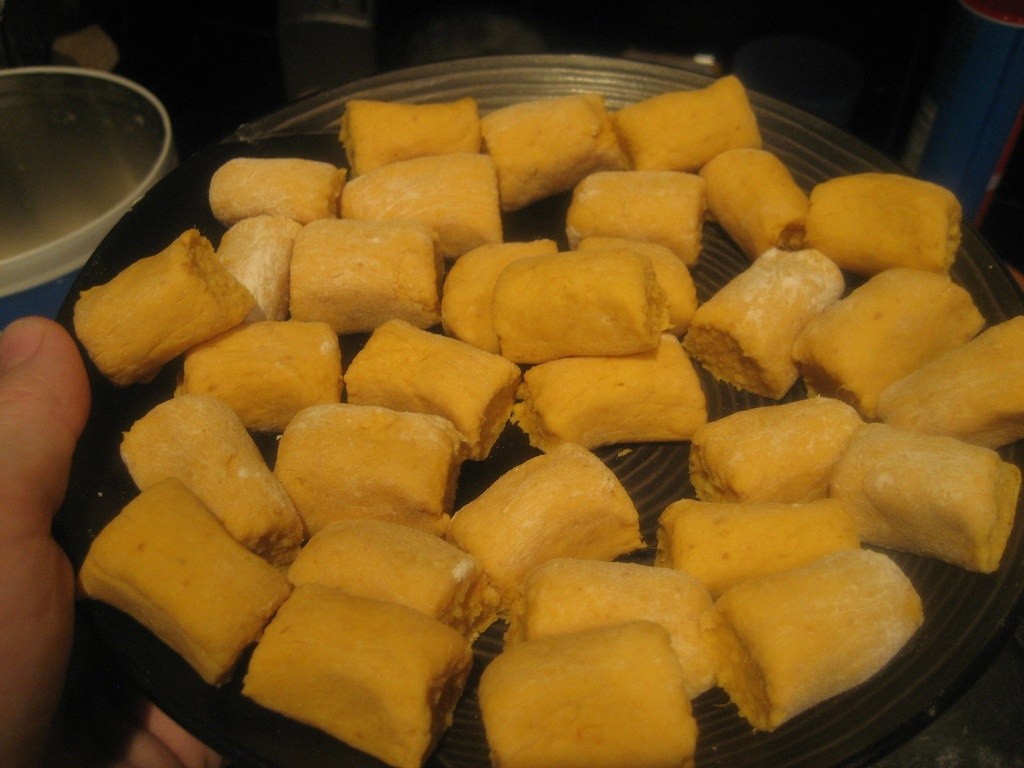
[0,66,179,334]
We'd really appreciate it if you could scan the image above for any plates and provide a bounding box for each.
[45,53,1024,768]
